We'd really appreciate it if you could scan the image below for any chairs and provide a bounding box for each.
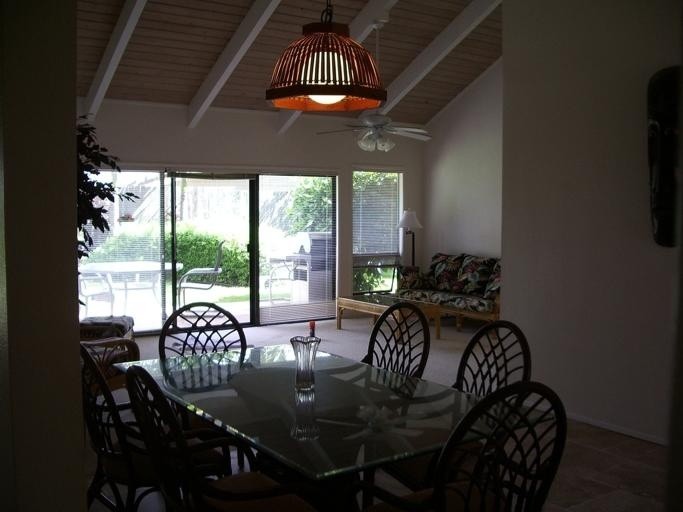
[350,380,566,512]
[317,302,430,414]
[78,344,317,511]
[159,302,255,392]
[79,273,114,317]
[175,240,224,307]
[362,319,531,512]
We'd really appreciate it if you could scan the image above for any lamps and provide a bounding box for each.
[397,208,422,265]
[265,0,387,112]
[357,128,395,152]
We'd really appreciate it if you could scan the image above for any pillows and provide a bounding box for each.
[454,253,496,297]
[424,253,464,292]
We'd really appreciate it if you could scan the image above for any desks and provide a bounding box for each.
[113,346,555,511]
[78,262,182,315]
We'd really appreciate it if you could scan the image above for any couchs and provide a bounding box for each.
[395,253,501,339]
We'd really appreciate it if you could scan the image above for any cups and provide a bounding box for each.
[289,334,321,393]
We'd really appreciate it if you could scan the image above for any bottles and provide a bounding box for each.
[308,320,315,337]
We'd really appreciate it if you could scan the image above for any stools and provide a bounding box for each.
[80,315,135,351]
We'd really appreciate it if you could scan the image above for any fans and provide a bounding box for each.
[314,109,432,141]
